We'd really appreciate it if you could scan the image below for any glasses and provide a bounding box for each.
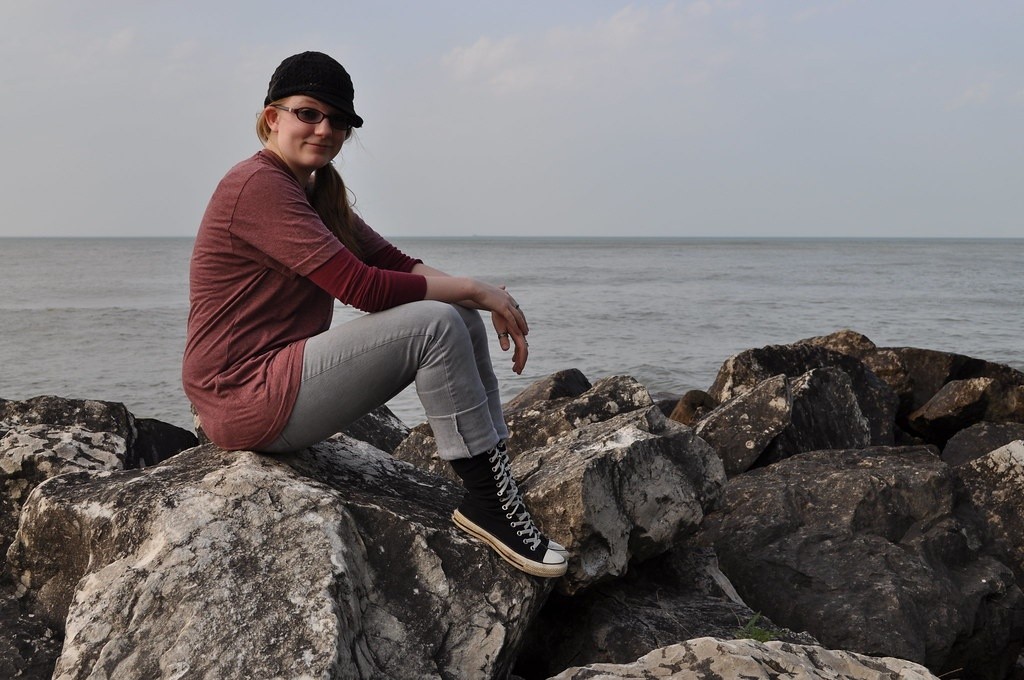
[274,104,353,131]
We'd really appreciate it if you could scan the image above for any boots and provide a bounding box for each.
[449,439,570,578]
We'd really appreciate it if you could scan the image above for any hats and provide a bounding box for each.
[268,50,363,128]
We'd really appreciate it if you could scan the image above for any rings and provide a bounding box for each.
[514,304,519,309]
[498,333,509,339]
[524,342,529,347]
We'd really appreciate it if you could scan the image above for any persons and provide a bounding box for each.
[183,51,571,577]
